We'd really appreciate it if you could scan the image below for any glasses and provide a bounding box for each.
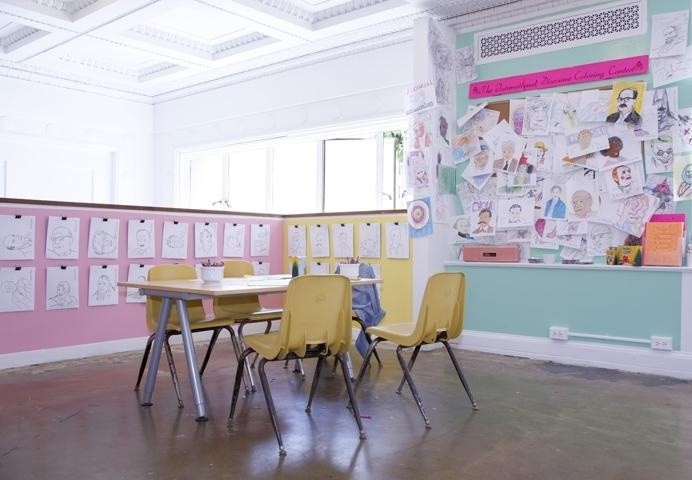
[656,148,672,155]
[617,96,636,102]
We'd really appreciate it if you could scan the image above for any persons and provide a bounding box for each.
[47,280,78,309]
[126,273,148,301]
[334,231,352,256]
[386,229,407,256]
[92,229,118,258]
[311,232,329,255]
[132,228,154,258]
[290,232,304,255]
[359,238,379,256]
[91,274,116,303]
[165,232,188,257]
[223,230,242,254]
[3,232,33,259]
[251,230,267,255]
[197,228,217,256]
[1,278,33,310]
[46,226,77,260]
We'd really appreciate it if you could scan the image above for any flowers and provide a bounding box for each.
[201,258,225,267]
[339,256,360,264]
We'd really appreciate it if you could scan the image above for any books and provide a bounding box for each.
[641,221,685,268]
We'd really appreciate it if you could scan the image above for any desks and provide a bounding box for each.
[116,273,385,422]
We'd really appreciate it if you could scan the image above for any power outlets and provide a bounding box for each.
[549,326,568,341]
[650,336,672,351]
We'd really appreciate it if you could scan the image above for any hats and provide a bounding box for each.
[533,142,547,151]
